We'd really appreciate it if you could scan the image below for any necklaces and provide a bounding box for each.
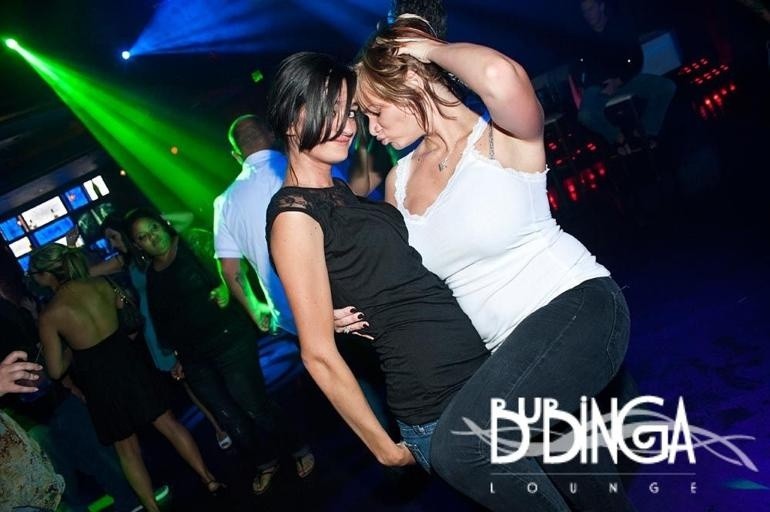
[422,136,470,172]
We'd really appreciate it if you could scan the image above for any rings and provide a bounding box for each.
[342,326,350,336]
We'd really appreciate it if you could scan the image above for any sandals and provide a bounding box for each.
[203,479,230,499]
[214,428,233,451]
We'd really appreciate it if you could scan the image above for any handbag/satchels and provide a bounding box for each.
[96,272,147,335]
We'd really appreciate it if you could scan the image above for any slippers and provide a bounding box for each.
[612,127,662,161]
[250,460,282,497]
[294,450,316,479]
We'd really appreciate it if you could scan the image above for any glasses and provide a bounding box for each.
[26,269,44,279]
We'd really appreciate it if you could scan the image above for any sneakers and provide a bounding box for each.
[128,484,171,512]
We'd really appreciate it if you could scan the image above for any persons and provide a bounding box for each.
[262,47,494,476]
[27,242,235,511]
[0,350,66,511]
[65,210,234,450]
[209,111,349,335]
[329,8,633,510]
[563,0,678,154]
[123,206,317,495]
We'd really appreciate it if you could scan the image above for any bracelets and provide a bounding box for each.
[68,245,76,248]
